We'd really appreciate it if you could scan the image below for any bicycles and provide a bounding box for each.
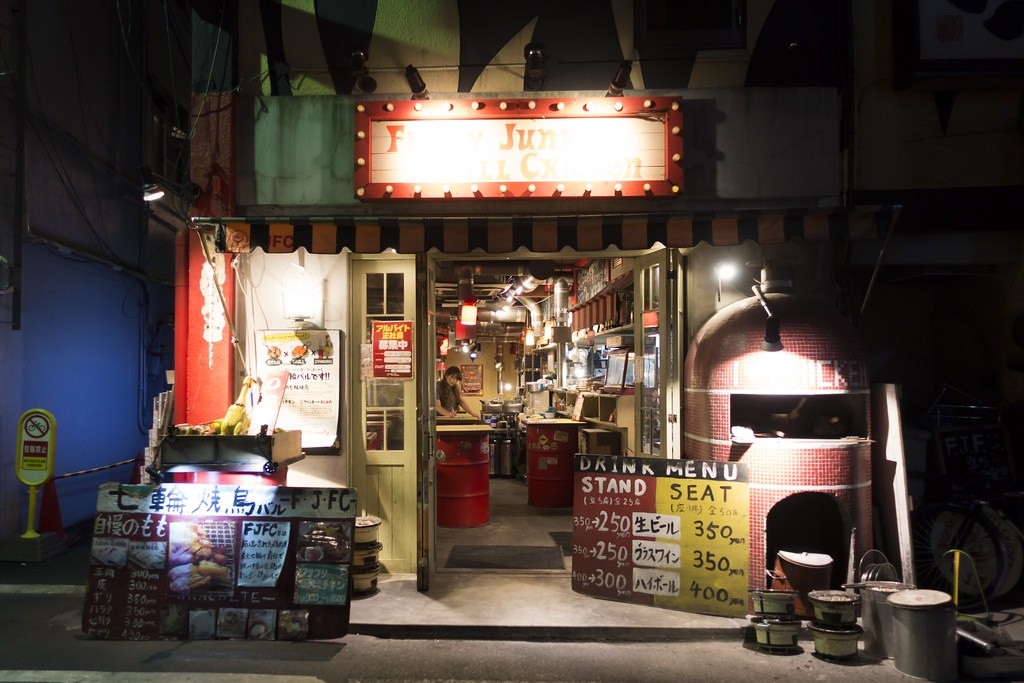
[910,444,1024,612]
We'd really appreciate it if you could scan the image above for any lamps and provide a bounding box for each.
[607,57,634,96]
[460,296,479,326]
[455,317,478,339]
[751,284,785,352]
[522,42,546,90]
[405,65,428,99]
[136,164,202,204]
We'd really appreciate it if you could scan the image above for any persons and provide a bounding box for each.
[434,366,487,418]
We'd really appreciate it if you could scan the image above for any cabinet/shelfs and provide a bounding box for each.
[551,323,637,459]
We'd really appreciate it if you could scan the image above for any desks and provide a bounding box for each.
[366,407,405,450]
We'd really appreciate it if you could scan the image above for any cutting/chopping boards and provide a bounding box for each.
[521,415,588,426]
[435,424,495,434]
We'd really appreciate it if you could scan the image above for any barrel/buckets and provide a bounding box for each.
[858,578,918,661]
[435,435,493,529]
[771,540,833,618]
[887,590,957,683]
[526,424,577,509]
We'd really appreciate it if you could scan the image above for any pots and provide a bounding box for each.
[806,621,863,657]
[747,589,799,616]
[807,590,861,625]
[750,617,802,646]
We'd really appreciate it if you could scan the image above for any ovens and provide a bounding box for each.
[604,350,659,395]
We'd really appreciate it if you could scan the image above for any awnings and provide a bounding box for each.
[191,194,839,255]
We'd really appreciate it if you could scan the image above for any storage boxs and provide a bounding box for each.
[580,429,622,456]
